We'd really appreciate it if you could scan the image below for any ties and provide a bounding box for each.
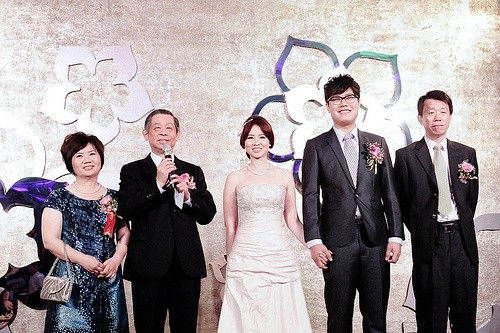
[344,133,360,217]
[433,144,454,217]
[164,174,173,191]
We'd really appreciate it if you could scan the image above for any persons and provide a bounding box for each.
[39,132,130,333]
[301,74,406,333]
[218,115,333,333]
[118,109,217,333]
[393,91,479,333]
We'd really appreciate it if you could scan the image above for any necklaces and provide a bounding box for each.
[247,163,272,175]
[73,181,100,194]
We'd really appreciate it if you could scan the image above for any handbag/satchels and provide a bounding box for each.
[40,243,73,303]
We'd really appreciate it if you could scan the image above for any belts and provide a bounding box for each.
[438,223,459,234]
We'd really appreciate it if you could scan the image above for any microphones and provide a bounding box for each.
[161,144,174,176]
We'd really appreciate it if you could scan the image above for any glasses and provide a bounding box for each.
[327,94,359,105]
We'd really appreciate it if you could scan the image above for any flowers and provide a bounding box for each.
[96,194,123,219]
[458,158,479,184]
[179,173,197,190]
[365,142,385,175]
